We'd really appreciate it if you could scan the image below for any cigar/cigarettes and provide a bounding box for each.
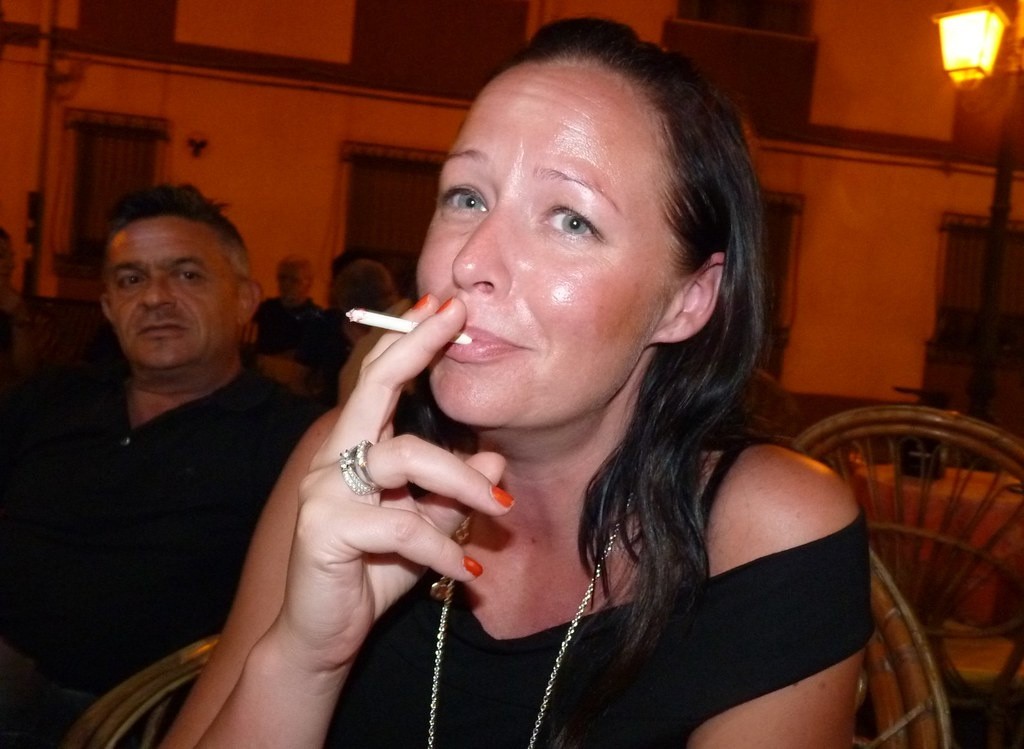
[346,309,472,345]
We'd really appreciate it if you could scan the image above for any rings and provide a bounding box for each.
[339,440,385,496]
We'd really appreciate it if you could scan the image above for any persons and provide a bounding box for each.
[0,185,416,749]
[157,17,872,749]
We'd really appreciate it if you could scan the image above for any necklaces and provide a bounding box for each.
[427,493,632,749]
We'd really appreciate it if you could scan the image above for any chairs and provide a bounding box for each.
[789,403,1024,749]
[848,547,954,749]
[60,632,224,749]
